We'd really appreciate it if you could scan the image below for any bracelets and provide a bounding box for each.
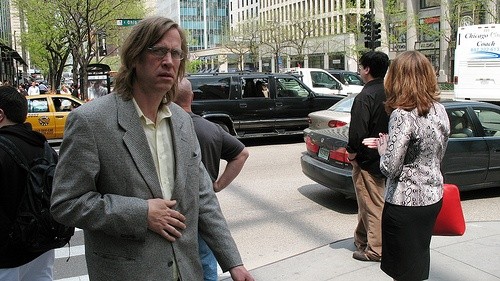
[348,157,356,161]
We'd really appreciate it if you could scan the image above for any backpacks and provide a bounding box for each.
[0,135,75,249]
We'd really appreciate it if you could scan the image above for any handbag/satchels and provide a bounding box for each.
[431,184,466,236]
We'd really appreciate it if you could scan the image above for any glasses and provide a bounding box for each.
[146,47,185,60]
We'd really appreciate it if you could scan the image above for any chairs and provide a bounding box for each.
[450,116,468,138]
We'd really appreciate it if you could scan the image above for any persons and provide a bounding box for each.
[346,51,390,261]
[60,86,72,107]
[71,83,78,98]
[49,16,255,281]
[0,86,56,281]
[172,77,249,281]
[462,114,472,135]
[362,51,450,281]
[19,81,47,96]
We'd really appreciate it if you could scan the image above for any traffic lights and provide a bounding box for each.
[370,21,382,41]
[364,11,372,49]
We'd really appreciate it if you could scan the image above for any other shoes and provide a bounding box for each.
[353,249,378,261]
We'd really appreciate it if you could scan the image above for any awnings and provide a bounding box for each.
[1,45,28,67]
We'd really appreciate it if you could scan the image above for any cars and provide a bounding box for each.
[24,94,88,140]
[302,93,361,144]
[187,71,345,140]
[300,101,500,201]
[281,67,367,96]
